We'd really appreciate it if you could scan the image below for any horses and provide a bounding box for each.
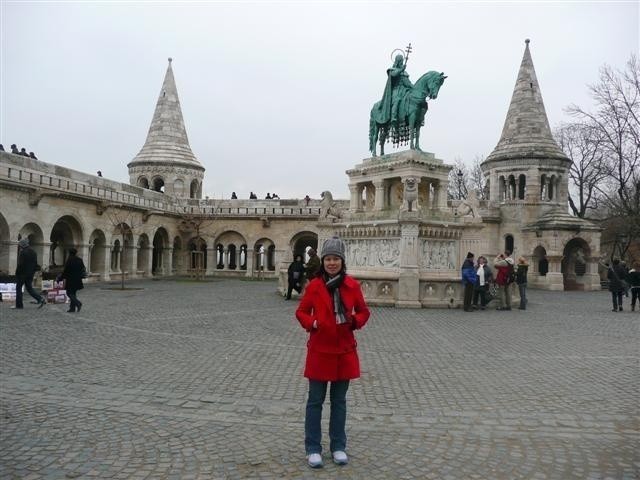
[369,70,448,158]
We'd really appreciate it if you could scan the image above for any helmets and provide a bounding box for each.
[467,251,474,258]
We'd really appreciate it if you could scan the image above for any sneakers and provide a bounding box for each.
[330,448,352,466]
[304,450,327,469]
[66,301,83,313]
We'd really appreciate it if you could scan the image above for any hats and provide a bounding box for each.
[320,237,346,263]
[18,237,31,249]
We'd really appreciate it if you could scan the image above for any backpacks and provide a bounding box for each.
[502,259,516,285]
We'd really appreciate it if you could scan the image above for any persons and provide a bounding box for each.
[607,258,627,312]
[621,261,628,297]
[56,246,86,312]
[381,54,426,126]
[285,253,303,300]
[303,248,321,282]
[231,192,237,199]
[513,256,529,310]
[624,259,640,311]
[474,256,493,309]
[265,193,272,199]
[461,251,480,311]
[492,249,517,311]
[296,237,371,468]
[97,171,102,177]
[250,192,257,199]
[272,194,277,199]
[9,238,46,310]
[304,195,311,199]
[0,142,38,159]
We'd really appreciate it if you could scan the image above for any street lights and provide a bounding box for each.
[457,168,463,201]
[618,233,628,260]
